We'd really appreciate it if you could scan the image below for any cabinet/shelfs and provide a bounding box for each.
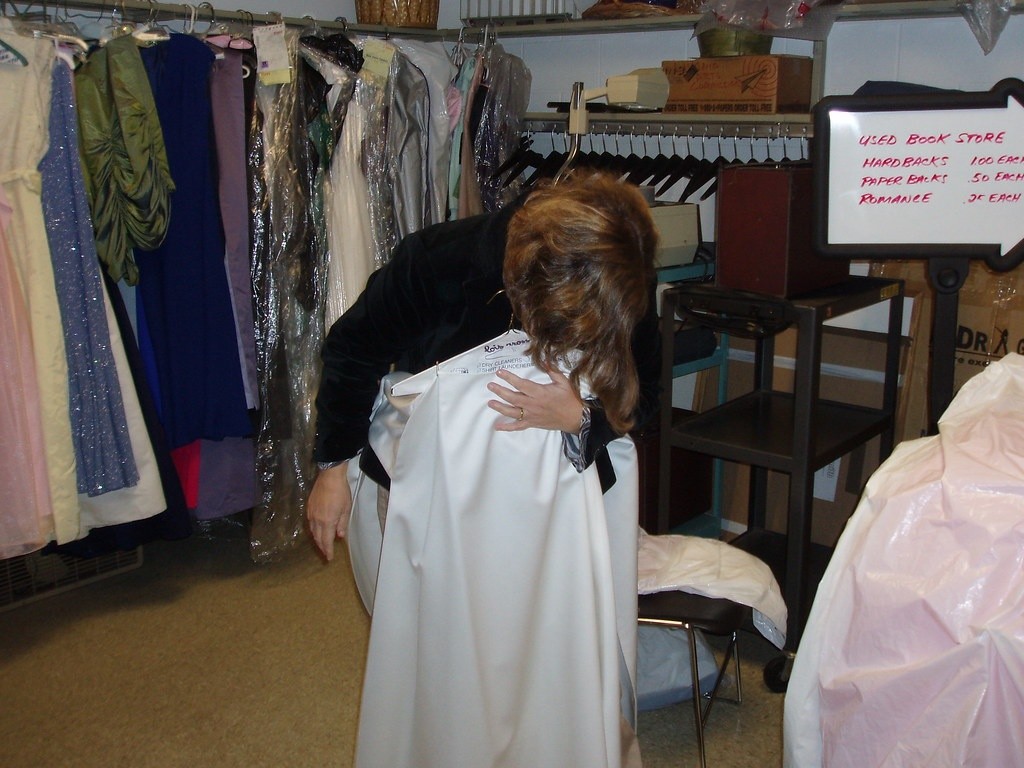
[655,278,905,686]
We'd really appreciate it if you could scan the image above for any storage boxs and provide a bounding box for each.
[694,261,1024,552]
[657,55,812,114]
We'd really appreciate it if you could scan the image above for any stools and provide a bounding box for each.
[636,546,742,768]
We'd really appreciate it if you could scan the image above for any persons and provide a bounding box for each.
[308,178,660,561]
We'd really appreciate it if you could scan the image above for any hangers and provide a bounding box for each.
[0,0,499,66]
[487,122,807,206]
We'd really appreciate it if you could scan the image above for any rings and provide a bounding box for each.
[518,408,523,420]
